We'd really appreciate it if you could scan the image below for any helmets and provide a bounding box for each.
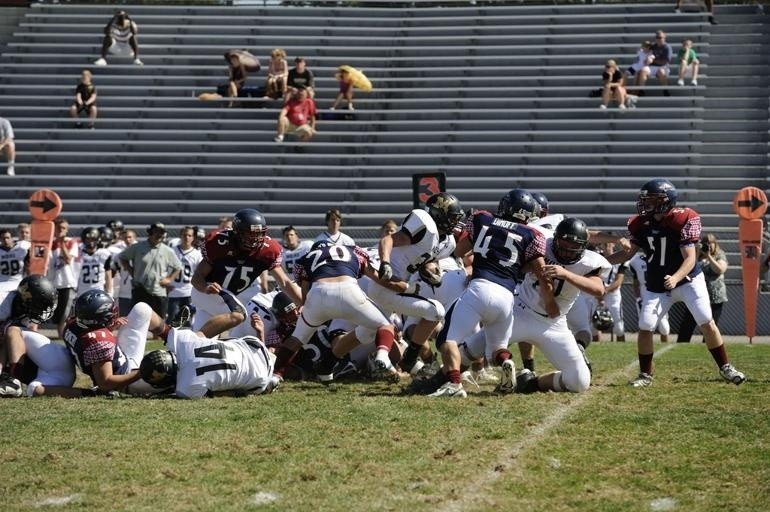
[637,178,679,222]
[497,189,536,225]
[531,190,550,217]
[270,290,301,331]
[193,226,207,247]
[80,227,101,249]
[96,226,113,246]
[140,349,178,393]
[104,219,124,238]
[232,208,267,254]
[591,305,615,332]
[14,274,59,326]
[423,191,465,238]
[74,289,118,330]
[552,216,590,266]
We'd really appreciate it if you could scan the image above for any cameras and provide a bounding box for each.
[701,242,711,253]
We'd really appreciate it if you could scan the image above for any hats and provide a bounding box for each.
[147,222,169,234]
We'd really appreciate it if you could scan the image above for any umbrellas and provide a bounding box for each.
[340,65,373,92]
[225,48,260,72]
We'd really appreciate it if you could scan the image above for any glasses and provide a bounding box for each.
[605,65,611,69]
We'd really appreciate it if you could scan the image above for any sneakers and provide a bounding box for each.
[6,165,17,177]
[500,357,517,394]
[471,366,500,384]
[719,362,747,386]
[329,361,358,380]
[522,377,548,394]
[273,135,284,142]
[93,58,107,65]
[312,353,339,384]
[599,104,607,110]
[374,350,400,385]
[627,371,655,388]
[348,103,354,111]
[133,58,144,65]
[576,343,592,378]
[0,377,23,399]
[517,370,536,392]
[265,373,283,396]
[399,351,441,387]
[618,105,626,110]
[677,78,685,87]
[426,380,468,400]
[459,370,480,389]
[690,80,699,86]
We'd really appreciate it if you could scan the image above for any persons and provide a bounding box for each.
[0,182,745,395]
[226,54,246,107]
[594,1,717,109]
[0,118,15,178]
[263,48,288,101]
[96,10,142,65]
[285,56,315,103]
[329,69,355,111]
[272,87,316,144]
[71,71,98,128]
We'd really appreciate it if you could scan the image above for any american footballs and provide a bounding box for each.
[418,261,439,285]
